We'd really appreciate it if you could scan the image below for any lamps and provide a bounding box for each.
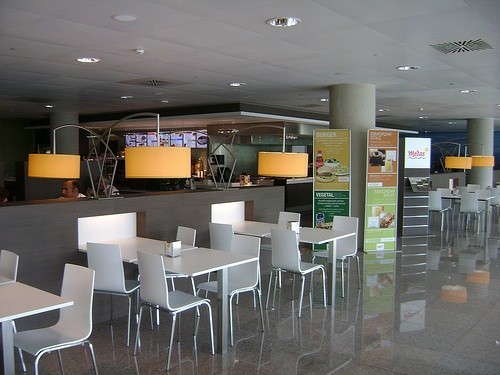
[432,141,473,170]
[207,124,309,190]
[85,112,192,200]
[28,123,118,200]
[440,143,495,173]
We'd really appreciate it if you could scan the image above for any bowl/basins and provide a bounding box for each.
[323,161,341,168]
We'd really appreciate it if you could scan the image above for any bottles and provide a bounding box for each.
[316,150,323,175]
[195,157,205,175]
[191,157,195,175]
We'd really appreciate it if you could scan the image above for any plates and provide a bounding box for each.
[330,168,350,175]
[197,136,207,145]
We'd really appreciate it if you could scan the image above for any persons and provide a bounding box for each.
[88,176,120,198]
[57,179,86,199]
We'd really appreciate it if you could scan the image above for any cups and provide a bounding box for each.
[286,220,300,234]
[370,151,383,165]
[165,240,182,258]
[385,158,392,171]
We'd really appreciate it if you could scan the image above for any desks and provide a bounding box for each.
[133,247,258,355]
[0,281,74,375]
[441,194,461,199]
[454,193,495,233]
[231,220,287,237]
[265,227,356,306]
[78,236,198,325]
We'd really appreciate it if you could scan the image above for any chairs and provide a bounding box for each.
[0,210,362,375]
[429,184,500,235]
